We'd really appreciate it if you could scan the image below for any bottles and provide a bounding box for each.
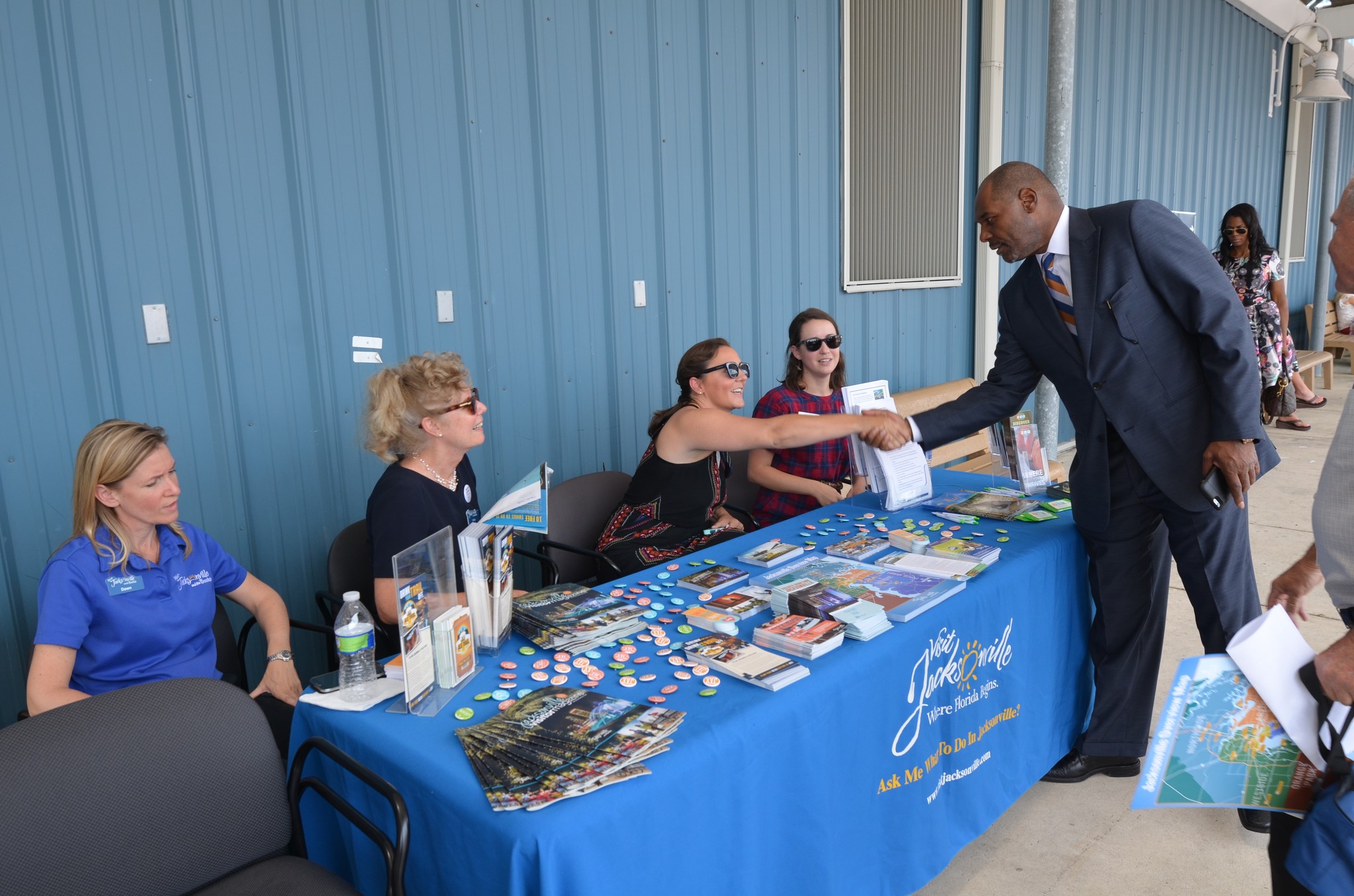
[923,449,931,481]
[334,591,379,703]
[1349,321,1354,336]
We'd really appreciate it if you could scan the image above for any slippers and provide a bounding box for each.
[1276,417,1311,431]
[1296,396,1327,409]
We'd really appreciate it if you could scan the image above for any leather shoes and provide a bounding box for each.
[1238,805,1272,834]
[1041,750,1142,783]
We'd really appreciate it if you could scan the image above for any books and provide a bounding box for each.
[510,582,646,657]
[920,489,1044,521]
[455,684,688,811]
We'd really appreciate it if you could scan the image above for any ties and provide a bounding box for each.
[1041,252,1079,346]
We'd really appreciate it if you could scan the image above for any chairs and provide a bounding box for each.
[537,471,633,589]
[0,677,410,896]
[17,596,335,723]
[722,450,761,533]
[315,518,559,673]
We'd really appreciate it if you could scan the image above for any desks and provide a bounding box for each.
[289,469,1096,896]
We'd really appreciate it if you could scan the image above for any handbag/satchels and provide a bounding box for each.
[1261,353,1297,425]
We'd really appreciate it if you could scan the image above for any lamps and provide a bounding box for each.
[1269,22,1351,118]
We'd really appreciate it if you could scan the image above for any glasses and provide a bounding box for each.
[796,335,842,352]
[419,387,480,429]
[1223,228,1248,235]
[693,362,750,378]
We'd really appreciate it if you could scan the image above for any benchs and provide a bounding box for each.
[1305,300,1354,375]
[890,377,1065,485]
[1295,350,1333,392]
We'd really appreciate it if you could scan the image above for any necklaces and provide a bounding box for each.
[411,452,459,493]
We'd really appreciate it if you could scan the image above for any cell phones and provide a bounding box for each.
[1199,464,1233,512]
[703,527,725,535]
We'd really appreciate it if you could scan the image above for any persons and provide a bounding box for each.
[747,308,866,533]
[858,160,1282,835]
[1333,292,1354,335]
[588,338,910,586]
[362,351,529,657]
[25,418,304,709]
[1212,202,1328,431]
[1266,179,1354,709]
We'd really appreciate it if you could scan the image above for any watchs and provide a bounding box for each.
[1241,438,1255,444]
[266,650,295,663]
